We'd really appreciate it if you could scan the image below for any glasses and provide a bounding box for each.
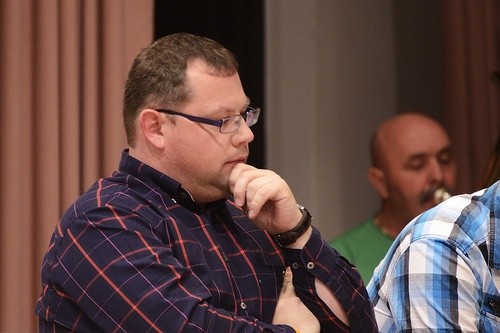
[154,106,261,134]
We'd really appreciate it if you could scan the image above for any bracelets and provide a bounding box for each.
[266,204,312,247]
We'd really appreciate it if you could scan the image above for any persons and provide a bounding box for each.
[365,179,499,333]
[34,33,380,333]
[330,111,456,287]
